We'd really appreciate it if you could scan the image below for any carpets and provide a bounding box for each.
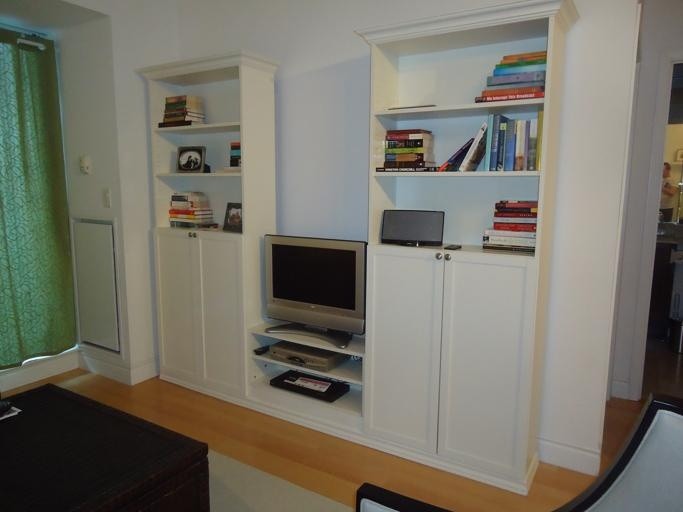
[205,449,355,512]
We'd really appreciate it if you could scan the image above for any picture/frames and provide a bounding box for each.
[673,148,683,163]
[222,201,242,233]
[175,144,205,173]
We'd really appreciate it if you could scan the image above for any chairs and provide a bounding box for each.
[354,391,682,512]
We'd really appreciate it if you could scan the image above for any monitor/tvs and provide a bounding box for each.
[264,234,367,349]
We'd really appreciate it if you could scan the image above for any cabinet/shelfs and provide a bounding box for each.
[246,318,367,438]
[133,49,282,234]
[364,247,555,496]
[155,228,279,406]
[355,1,581,258]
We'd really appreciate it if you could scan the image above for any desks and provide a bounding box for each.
[0,381,211,512]
[646,232,678,341]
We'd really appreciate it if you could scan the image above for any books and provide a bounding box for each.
[482,200,538,255]
[474,51,547,103]
[376,108,544,172]
[229,141,240,167]
[169,191,218,229]
[158,95,208,128]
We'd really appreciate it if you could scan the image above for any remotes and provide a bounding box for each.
[254,346,269,355]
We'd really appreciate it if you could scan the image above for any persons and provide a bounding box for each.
[658,161,678,223]
[184,154,198,168]
[228,211,241,223]
